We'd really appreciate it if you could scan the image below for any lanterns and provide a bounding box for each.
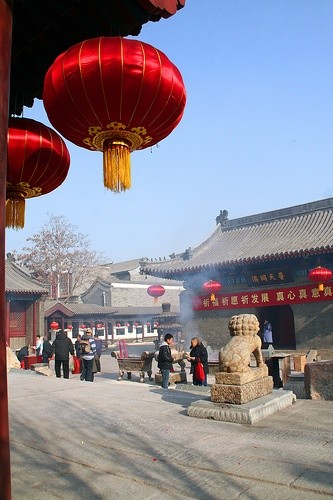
[50,320,160,330]
[6,117,70,230]
[308,266,333,292]
[202,280,222,302]
[43,36,186,195]
[146,285,165,304]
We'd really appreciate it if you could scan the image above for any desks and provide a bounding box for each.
[24,354,43,370]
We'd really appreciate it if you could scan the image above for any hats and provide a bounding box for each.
[86,328,92,333]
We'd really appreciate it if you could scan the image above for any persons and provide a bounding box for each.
[33,335,42,355]
[42,336,51,363]
[48,330,74,379]
[185,337,209,387]
[157,334,177,389]
[75,329,102,382]
[18,347,27,361]
[263,319,273,349]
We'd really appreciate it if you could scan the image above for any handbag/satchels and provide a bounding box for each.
[195,363,205,381]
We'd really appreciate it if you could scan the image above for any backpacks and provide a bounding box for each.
[79,338,90,354]
